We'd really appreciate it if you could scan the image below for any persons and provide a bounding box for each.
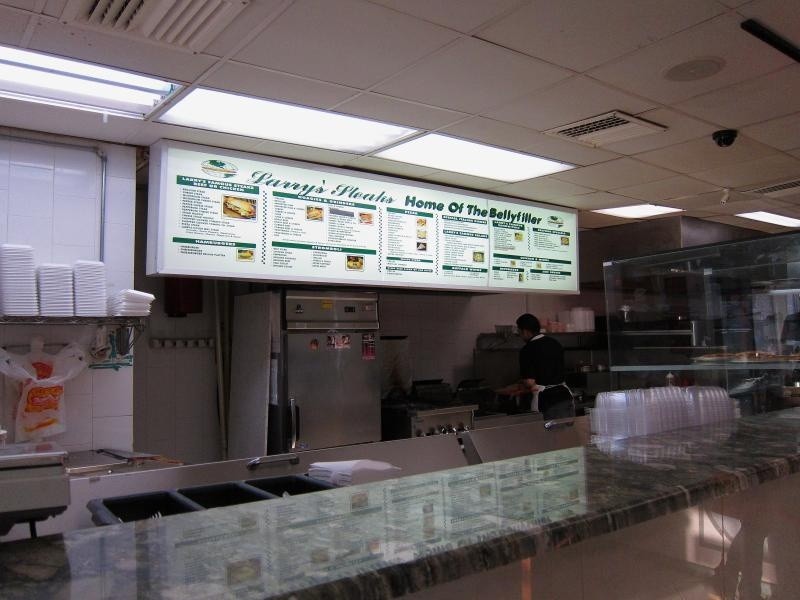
[501,312,578,419]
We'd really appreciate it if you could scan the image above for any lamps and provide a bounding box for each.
[0,45,174,108]
